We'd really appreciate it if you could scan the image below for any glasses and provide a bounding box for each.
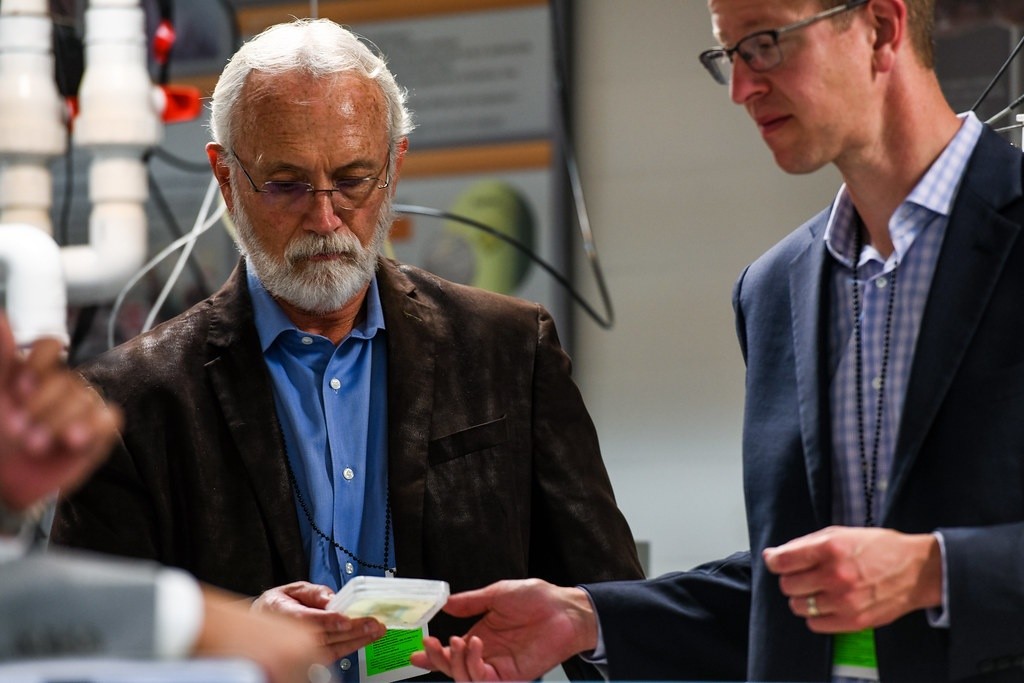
[231,123,392,209]
[698,0,867,86]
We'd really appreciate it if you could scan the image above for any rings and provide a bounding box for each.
[807,597,817,616]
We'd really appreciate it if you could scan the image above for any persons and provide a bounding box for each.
[411,1,1024,683]
[0,300,335,681]
[20,17,647,682]
